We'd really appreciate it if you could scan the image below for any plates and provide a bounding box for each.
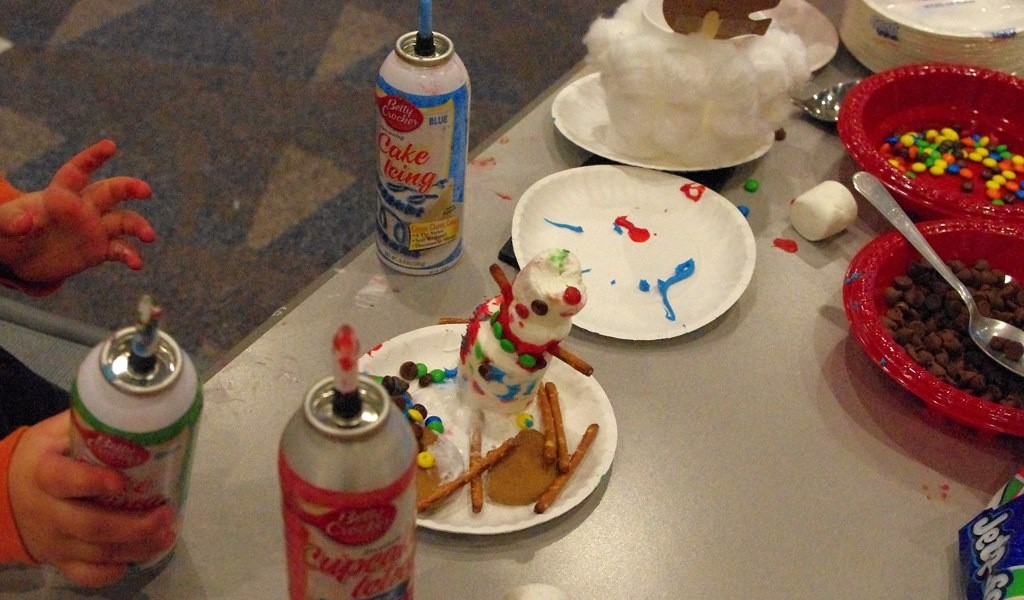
[642,0,839,72]
[551,72,775,172]
[512,165,756,340]
[839,0,1024,81]
[359,323,617,535]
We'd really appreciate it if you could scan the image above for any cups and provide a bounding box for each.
[663,0,781,40]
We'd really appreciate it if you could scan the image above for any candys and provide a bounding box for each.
[379,361,445,470]
[880,126,1023,209]
[735,180,757,218]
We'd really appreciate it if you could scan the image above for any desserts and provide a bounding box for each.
[457,248,594,413]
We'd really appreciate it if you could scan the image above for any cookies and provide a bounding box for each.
[409,380,599,516]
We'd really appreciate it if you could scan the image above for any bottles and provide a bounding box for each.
[68,295,202,576]
[374,0,469,276]
[278,325,417,600]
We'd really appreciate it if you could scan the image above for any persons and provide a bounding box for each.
[0,141,180,586]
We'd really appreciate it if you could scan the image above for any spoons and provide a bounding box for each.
[786,79,865,122]
[853,171,1024,377]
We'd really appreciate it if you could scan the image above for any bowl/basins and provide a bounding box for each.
[839,63,1024,225]
[844,219,1024,436]
